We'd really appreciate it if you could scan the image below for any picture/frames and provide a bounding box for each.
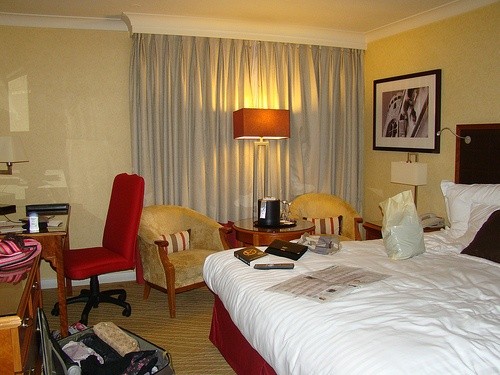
[373,69,441,154]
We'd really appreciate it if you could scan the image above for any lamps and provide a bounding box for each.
[391,154,425,186]
[233,109,290,198]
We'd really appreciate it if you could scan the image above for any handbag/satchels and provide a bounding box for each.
[379,190,426,261]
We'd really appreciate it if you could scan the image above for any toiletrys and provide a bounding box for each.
[28,212,40,234]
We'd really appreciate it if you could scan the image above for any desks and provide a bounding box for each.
[0,206,73,337]
[233,216,315,249]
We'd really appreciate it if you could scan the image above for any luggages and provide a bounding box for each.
[37,308,175,375]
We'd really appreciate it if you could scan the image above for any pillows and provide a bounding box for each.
[460,209,500,263]
[303,216,343,235]
[438,180,500,235]
[454,204,500,247]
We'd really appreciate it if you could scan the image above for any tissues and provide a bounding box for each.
[257,196,280,229]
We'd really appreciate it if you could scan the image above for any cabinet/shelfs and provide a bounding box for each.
[0,239,44,375]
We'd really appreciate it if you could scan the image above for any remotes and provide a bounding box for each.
[254,263,294,269]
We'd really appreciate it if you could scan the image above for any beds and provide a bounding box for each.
[203,123,500,375]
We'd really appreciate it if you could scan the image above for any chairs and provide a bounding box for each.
[52,172,146,328]
[287,192,364,242]
[137,204,232,317]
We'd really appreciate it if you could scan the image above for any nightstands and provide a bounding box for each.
[362,216,444,239]
